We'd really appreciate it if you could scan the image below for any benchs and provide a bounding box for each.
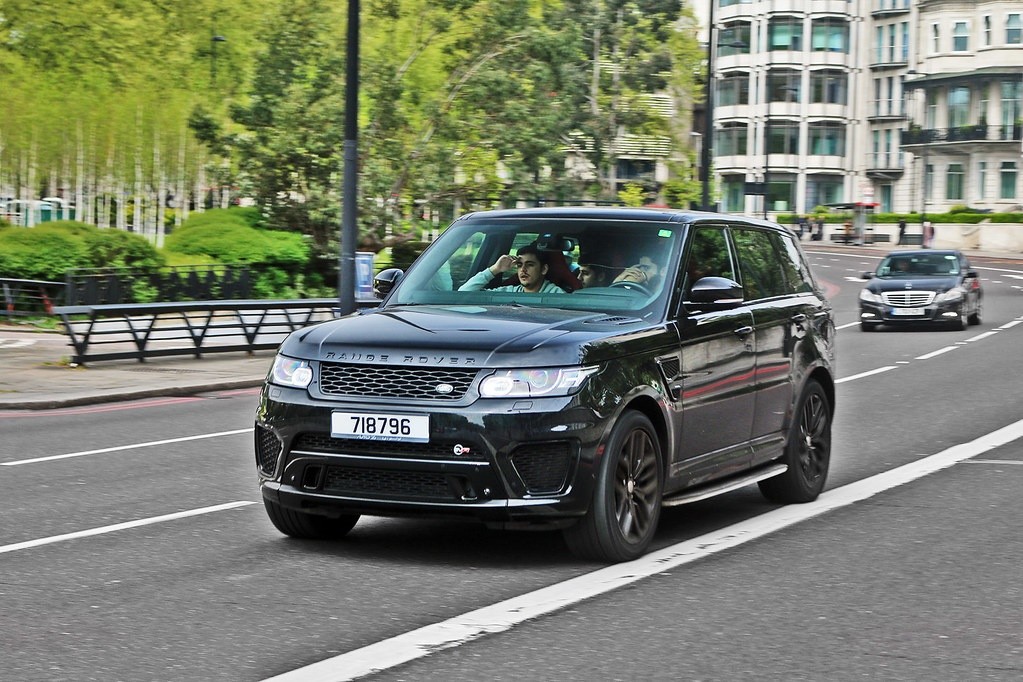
[53,295,390,368]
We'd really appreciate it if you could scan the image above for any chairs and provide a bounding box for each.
[501,236,583,293]
[929,256,954,273]
[891,256,920,273]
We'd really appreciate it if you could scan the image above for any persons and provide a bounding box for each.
[421,260,453,291]
[896,259,910,271]
[612,256,667,292]
[576,255,607,287]
[457,245,567,293]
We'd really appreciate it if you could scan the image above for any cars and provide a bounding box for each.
[860,250,987,331]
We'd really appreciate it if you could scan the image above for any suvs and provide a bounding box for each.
[254,209,839,563]
